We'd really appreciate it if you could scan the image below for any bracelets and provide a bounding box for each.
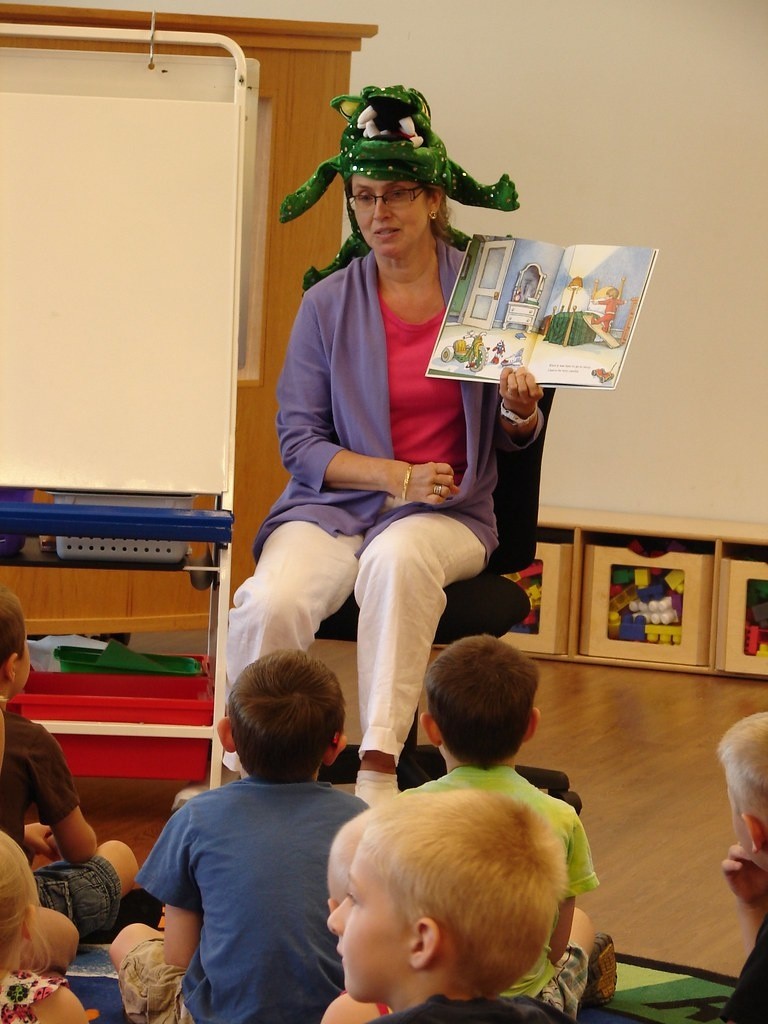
[400,462,413,502]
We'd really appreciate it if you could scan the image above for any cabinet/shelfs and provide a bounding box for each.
[0,484,235,816]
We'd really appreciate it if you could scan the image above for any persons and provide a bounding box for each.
[319,811,397,1023]
[390,631,621,1023]
[110,644,372,1024]
[218,142,545,810]
[0,829,88,1024]
[713,708,768,1023]
[322,786,572,1023]
[0,584,163,953]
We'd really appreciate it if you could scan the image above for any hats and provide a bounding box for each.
[280,85,518,297]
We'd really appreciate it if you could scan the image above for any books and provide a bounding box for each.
[425,232,660,392]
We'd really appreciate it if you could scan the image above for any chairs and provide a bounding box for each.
[310,383,584,827]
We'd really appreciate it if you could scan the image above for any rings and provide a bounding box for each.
[432,484,443,496]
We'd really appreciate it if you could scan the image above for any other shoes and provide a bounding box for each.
[583,932,617,1006]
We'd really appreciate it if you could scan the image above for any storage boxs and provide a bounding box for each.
[497,506,768,681]
[6,646,214,784]
[45,489,200,574]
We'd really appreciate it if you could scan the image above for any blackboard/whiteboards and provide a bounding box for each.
[0,45,247,497]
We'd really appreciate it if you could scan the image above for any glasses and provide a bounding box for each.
[346,185,430,209]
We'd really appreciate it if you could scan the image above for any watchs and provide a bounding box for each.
[499,397,539,426]
